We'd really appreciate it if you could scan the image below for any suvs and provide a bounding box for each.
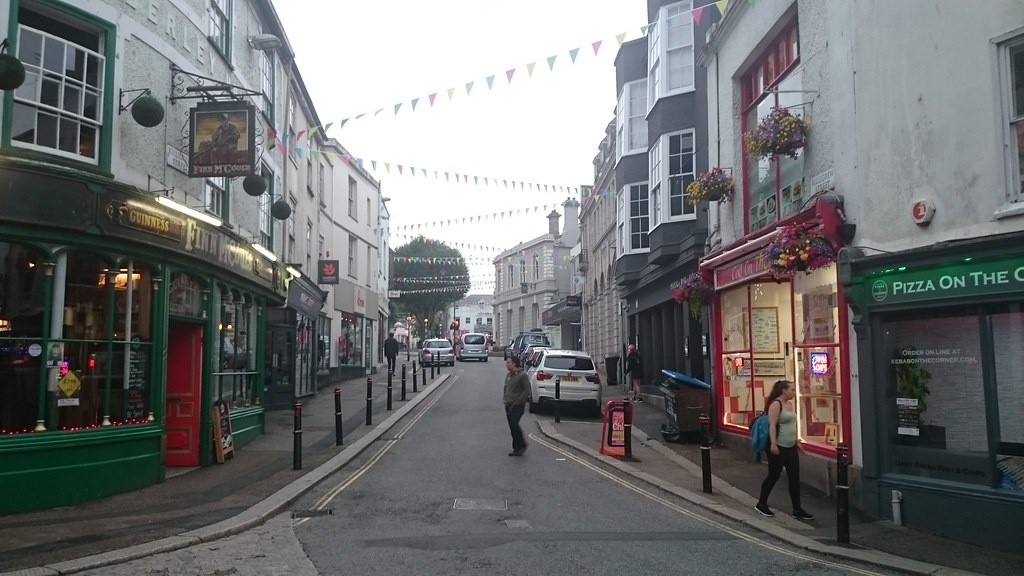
[513,332,550,359]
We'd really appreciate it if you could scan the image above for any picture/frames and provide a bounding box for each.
[743,306,780,353]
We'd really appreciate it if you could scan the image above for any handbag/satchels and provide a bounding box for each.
[748,399,782,451]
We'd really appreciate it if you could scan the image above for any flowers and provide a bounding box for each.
[762,226,828,284]
[673,274,714,318]
[744,110,806,160]
[687,166,731,207]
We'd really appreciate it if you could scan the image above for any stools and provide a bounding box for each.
[824,422,839,447]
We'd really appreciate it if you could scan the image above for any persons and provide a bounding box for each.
[623,343,643,402]
[502,356,531,456]
[383,332,399,376]
[211,113,240,165]
[754,379,814,519]
[318,333,326,370]
[342,333,353,364]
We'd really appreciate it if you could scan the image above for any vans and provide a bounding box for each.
[455,333,488,362]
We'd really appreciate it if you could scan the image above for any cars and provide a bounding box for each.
[520,344,553,373]
[418,338,455,367]
[526,349,601,415]
[503,340,515,361]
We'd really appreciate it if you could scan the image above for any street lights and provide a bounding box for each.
[439,324,442,338]
[424,319,428,339]
[407,317,412,360]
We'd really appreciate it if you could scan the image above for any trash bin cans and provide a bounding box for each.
[656,369,712,441]
[398,342,403,350]
[602,353,626,386]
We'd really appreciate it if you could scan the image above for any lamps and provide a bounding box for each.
[248,34,282,53]
[286,264,302,278]
[250,242,277,263]
[155,195,222,227]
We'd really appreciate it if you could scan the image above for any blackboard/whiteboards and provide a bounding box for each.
[211,401,233,451]
[897,404,919,428]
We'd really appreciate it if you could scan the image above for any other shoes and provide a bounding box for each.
[509,442,529,457]
[631,396,643,403]
[392,373,396,376]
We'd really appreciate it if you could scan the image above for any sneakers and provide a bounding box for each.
[792,508,815,520]
[754,501,775,517]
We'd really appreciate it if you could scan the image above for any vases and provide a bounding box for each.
[794,258,808,271]
[771,141,800,158]
[706,186,722,201]
